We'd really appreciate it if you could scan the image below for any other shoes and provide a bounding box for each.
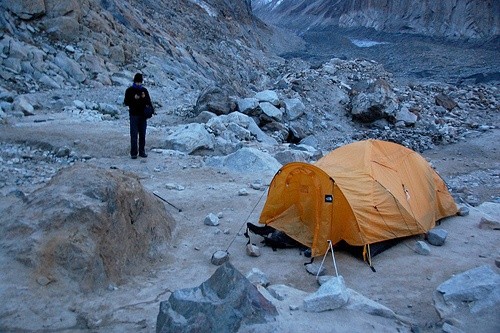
[139,153,147,158]
[131,155,137,159]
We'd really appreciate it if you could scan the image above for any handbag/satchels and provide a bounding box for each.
[145,104,152,117]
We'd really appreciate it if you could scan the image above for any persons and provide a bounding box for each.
[124,73,154,159]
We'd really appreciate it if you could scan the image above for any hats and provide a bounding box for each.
[133,73,143,83]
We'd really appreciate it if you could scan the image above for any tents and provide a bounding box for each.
[225,139,468,280]
